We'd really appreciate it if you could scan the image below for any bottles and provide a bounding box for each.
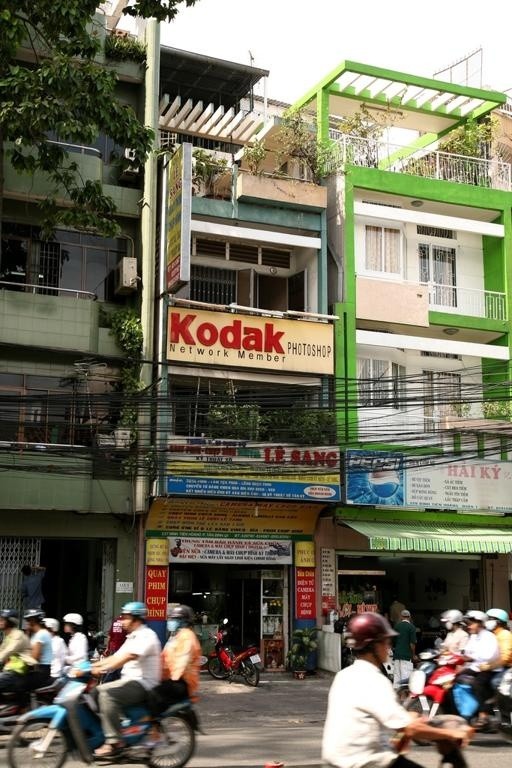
[268,600,283,614]
[263,617,280,633]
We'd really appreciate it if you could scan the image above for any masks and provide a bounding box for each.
[444,622,453,630]
[166,619,181,631]
[484,620,497,631]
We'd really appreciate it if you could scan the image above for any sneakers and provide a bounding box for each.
[475,716,489,727]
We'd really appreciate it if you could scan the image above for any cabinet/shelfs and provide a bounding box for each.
[258,576,285,672]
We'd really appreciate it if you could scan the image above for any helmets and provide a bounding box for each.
[120,602,148,618]
[440,608,509,623]
[0,608,84,632]
[167,605,193,619]
[342,611,400,652]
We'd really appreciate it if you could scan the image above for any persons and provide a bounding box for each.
[392,609,512,728]
[321,611,471,768]
[0,563,205,757]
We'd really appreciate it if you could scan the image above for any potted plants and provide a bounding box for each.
[287,643,309,680]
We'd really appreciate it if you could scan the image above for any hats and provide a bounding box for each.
[401,610,410,616]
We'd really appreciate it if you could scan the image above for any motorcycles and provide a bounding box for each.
[0,686,51,744]
[404,651,512,745]
[395,648,439,700]
[264,715,475,768]
[88,629,112,662]
[7,659,207,767]
[207,617,261,686]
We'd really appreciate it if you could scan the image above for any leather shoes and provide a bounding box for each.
[94,741,124,755]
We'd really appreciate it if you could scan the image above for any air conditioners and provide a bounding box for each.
[119,147,139,180]
[111,428,133,451]
[115,256,139,298]
